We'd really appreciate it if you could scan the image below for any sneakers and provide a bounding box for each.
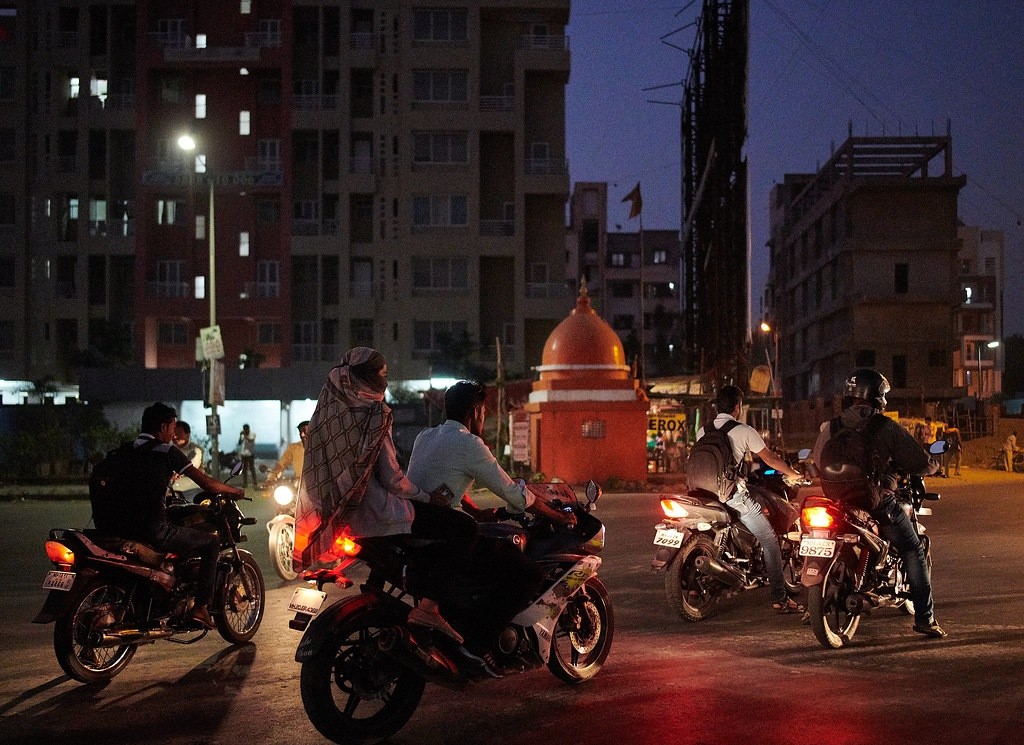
[460,645,506,679]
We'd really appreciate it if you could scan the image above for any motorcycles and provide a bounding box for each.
[207,447,240,473]
[798,438,952,650]
[254,463,300,582]
[288,475,615,744]
[651,447,813,624]
[31,460,266,686]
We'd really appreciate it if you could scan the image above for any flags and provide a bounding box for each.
[623,186,641,219]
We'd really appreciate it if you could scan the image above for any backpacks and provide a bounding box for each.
[89,441,170,540]
[686,421,743,504]
[821,415,890,510]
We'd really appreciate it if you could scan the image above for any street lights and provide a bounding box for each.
[979,340,1000,438]
[178,136,222,483]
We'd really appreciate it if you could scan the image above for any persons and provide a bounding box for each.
[697,386,804,612]
[651,430,667,450]
[1003,431,1018,472]
[265,421,310,483]
[305,346,478,643]
[914,417,963,476]
[239,425,258,488]
[814,370,947,636]
[167,421,203,503]
[405,383,578,678]
[134,402,244,630]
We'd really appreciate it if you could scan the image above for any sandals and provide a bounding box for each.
[773,592,805,614]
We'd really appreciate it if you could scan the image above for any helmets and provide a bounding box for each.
[843,369,890,411]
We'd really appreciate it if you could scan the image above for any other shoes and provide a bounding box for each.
[190,607,217,629]
[914,620,948,639]
[407,608,464,644]
